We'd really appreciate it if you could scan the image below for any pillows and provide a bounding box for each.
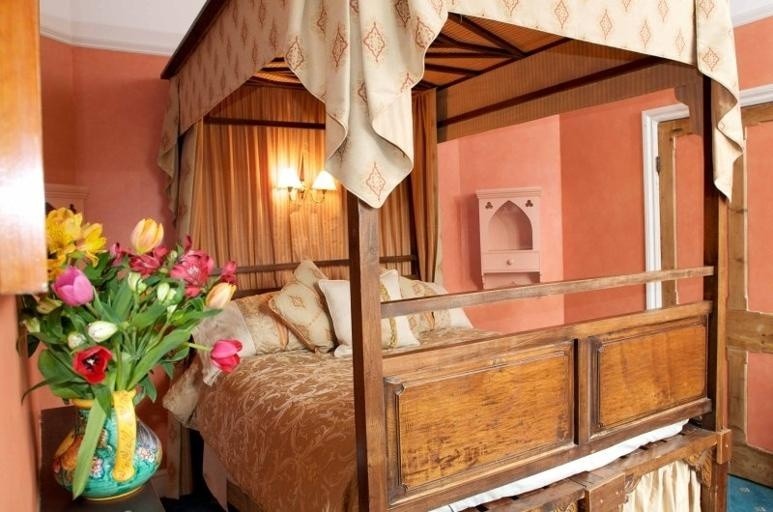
[380,267,474,339]
[191,287,307,387]
[269,259,334,359]
[318,269,420,358]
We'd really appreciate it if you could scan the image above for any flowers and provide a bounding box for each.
[16,201,245,501]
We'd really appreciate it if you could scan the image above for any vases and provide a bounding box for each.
[52,399,164,500]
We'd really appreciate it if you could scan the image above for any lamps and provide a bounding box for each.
[274,168,338,203]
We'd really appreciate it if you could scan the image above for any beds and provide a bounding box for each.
[154,253,741,511]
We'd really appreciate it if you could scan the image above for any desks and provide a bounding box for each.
[38,406,170,512]
